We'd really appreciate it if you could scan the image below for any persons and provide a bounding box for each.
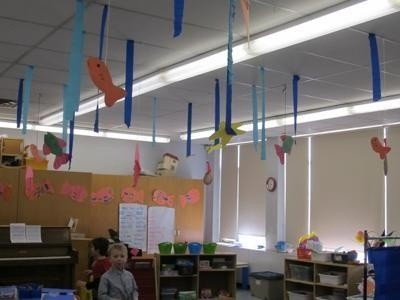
[97,242,139,300]
[75,237,111,299]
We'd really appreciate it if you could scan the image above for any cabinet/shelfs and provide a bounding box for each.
[283,256,370,300]
[154,252,238,300]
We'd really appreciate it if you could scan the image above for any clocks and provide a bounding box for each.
[266,177,275,191]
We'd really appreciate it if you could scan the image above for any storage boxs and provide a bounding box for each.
[249,271,283,300]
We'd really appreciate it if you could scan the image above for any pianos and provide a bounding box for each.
[0,225,79,290]
